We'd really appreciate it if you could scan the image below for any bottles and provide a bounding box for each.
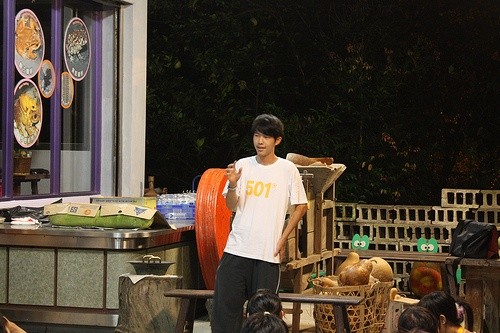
[156,192,196,224]
[144,176,157,197]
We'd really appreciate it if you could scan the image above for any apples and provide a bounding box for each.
[409,266,442,297]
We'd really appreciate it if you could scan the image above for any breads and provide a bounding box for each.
[15,15,41,59]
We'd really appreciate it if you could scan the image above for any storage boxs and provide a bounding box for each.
[13,157,32,176]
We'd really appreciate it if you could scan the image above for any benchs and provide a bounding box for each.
[335,252,458,302]
[164,290,365,333]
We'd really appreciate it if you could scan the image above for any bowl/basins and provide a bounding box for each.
[13,158,32,172]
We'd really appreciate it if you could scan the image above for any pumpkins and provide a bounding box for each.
[309,249,400,333]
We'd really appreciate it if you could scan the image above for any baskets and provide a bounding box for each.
[312,276,393,333]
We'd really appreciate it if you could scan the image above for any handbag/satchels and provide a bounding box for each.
[445,219,500,258]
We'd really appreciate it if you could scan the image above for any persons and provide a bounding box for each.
[210,114,308,333]
[1,289,481,332]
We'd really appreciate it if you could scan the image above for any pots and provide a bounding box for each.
[127,255,176,275]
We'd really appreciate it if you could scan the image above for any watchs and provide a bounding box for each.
[227,185,237,191]
